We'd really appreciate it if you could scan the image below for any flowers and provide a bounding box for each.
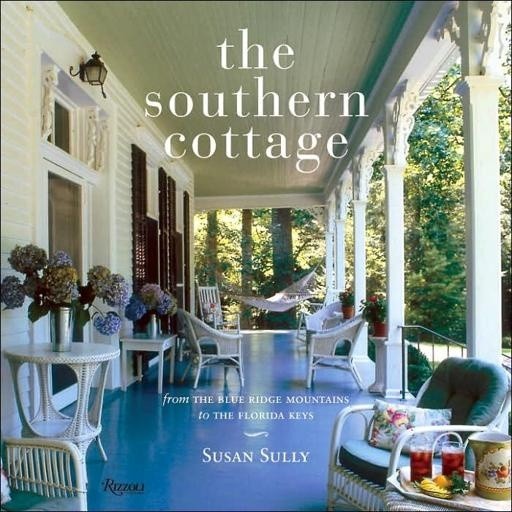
[0,240,128,336]
[122,281,178,321]
[359,293,387,323]
[335,286,355,307]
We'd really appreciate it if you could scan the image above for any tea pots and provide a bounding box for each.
[468,433,512,502]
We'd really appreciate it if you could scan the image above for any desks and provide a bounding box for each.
[5,340,121,484]
[119,332,178,394]
[377,483,476,512]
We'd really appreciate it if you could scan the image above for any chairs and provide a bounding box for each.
[302,301,343,352]
[305,312,365,391]
[323,354,510,512]
[176,308,246,389]
[194,281,241,336]
[295,283,340,343]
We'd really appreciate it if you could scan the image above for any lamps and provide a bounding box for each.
[70,47,112,98]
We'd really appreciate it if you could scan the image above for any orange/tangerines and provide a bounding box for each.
[433,475,449,486]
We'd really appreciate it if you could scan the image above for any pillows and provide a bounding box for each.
[368,397,453,456]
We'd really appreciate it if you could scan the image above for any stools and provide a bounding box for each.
[0,432,91,511]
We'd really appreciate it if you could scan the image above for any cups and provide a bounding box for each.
[442,442,464,482]
[409,438,432,482]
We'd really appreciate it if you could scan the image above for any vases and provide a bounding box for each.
[49,306,74,352]
[374,322,386,336]
[145,314,162,338]
[341,304,353,319]
